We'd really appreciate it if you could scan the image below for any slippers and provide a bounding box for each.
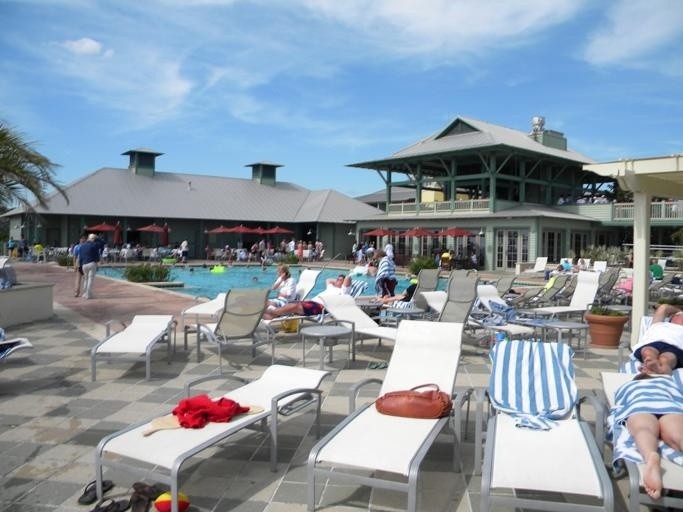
[78,480,111,505]
[90,498,130,512]
[133,483,159,499]
[130,486,151,512]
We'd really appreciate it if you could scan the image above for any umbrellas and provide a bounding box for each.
[83,221,172,249]
[363,226,476,251]
[207,224,295,246]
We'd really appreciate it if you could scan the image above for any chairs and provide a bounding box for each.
[86,310,177,386]
[0,336,33,358]
[178,250,680,377]
[304,317,475,508]
[92,361,332,508]
[472,338,618,508]
[578,316,680,505]
[9,234,326,263]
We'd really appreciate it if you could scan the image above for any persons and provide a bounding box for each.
[557,192,618,205]
[203,237,324,274]
[351,237,395,277]
[561,260,571,271]
[614,373,683,500]
[252,276,259,283]
[650,258,664,281]
[632,304,683,376]
[469,195,488,207]
[373,249,396,323]
[260,275,352,320]
[266,265,296,307]
[383,278,419,304]
[633,423,634,427]
[577,259,587,270]
[7,233,193,296]
[434,245,478,269]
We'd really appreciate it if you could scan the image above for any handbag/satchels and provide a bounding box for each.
[375,384,451,419]
